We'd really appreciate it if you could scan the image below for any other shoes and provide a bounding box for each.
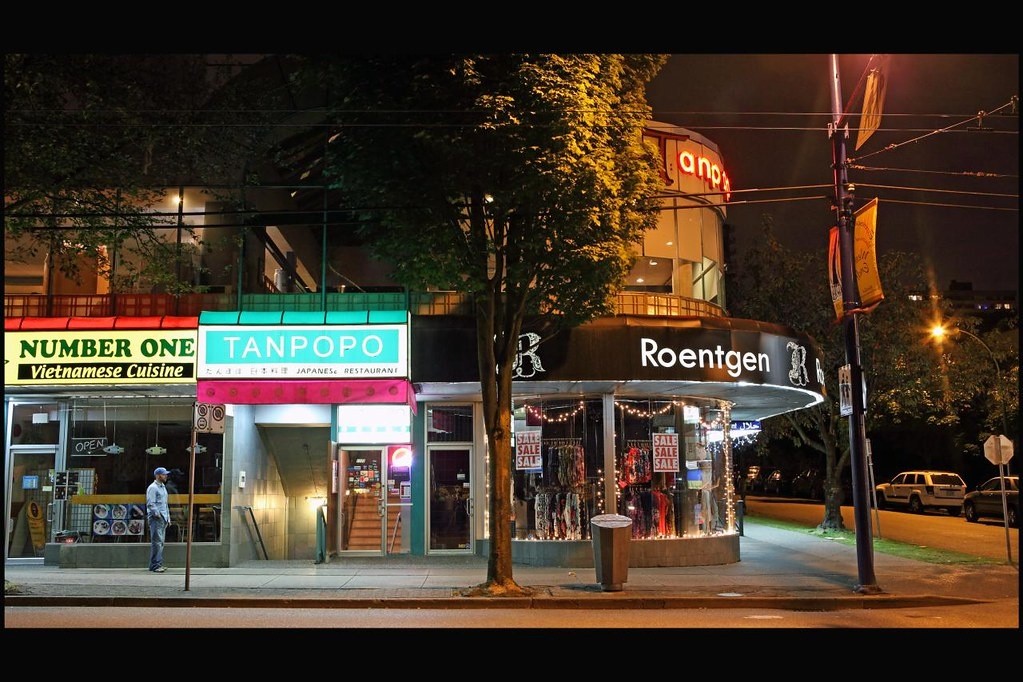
[161,566,167,570]
[154,568,164,572]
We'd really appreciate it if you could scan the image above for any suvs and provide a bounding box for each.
[870,470,966,517]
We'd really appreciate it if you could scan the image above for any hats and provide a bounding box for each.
[154,467,170,475]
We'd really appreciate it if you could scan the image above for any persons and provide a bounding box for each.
[165,469,184,542]
[146,468,171,572]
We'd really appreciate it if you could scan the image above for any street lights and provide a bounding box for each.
[933,327,1010,478]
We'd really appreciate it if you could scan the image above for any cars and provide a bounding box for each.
[963,477,1019,527]
[733,464,825,500]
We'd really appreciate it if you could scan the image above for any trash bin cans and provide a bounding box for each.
[590,514,633,592]
